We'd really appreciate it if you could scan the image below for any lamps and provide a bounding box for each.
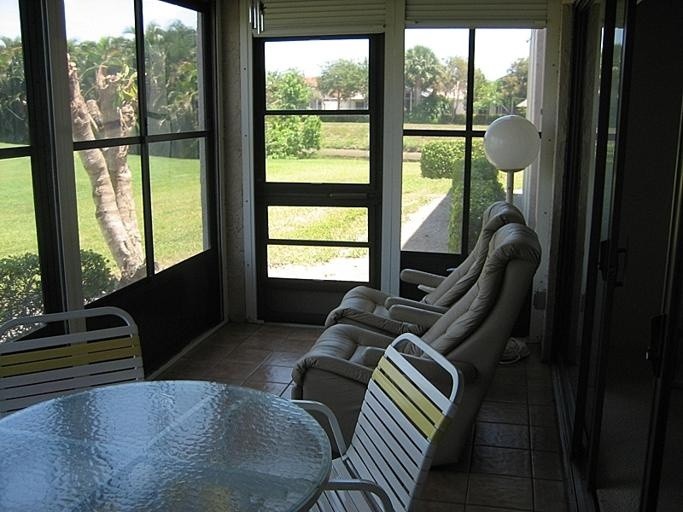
[481,113,541,363]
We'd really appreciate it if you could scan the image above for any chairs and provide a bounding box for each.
[0,307,145,418]
[289,223,544,467]
[321,199,525,335]
[285,331,463,510]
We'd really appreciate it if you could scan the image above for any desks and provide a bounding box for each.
[0,378,335,511]
